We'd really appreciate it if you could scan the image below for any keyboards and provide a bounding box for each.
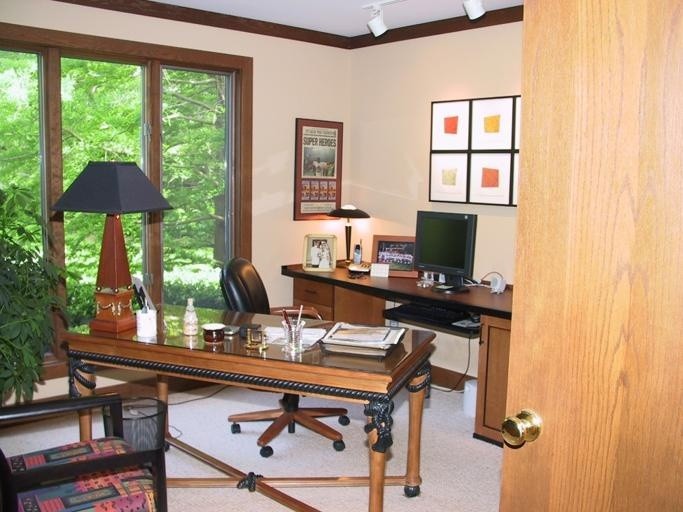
[386,302,469,326]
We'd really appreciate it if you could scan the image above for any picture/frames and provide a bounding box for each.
[302,232,338,275]
[427,93,522,208]
[292,116,344,223]
[370,233,418,280]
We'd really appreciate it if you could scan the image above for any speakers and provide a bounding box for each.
[491,276,506,294]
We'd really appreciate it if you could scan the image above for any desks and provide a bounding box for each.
[280,255,513,451]
[59,304,438,511]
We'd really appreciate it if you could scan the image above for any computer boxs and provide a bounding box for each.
[464,379,477,418]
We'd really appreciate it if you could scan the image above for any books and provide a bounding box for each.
[317,320,408,362]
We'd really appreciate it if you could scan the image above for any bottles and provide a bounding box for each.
[183,299,197,337]
[352,245,361,266]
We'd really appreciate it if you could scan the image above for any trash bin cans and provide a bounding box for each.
[102,396,167,455]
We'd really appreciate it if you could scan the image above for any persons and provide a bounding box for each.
[379,251,412,265]
[319,239,330,267]
[304,153,335,177]
[310,240,321,267]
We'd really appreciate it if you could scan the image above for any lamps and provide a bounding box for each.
[361,1,488,39]
[325,204,373,271]
[50,158,175,335]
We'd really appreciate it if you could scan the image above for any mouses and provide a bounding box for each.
[472,314,480,323]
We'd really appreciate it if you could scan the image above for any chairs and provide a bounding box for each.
[219,256,352,458]
[0,393,170,510]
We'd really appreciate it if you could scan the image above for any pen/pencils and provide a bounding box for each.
[296,304,304,340]
[282,308,293,344]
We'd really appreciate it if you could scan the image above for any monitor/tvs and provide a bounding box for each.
[413,211,478,293]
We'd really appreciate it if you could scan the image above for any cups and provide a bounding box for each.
[134,309,157,339]
[200,322,226,344]
[280,319,305,355]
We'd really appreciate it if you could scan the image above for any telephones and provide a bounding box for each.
[348,239,371,273]
